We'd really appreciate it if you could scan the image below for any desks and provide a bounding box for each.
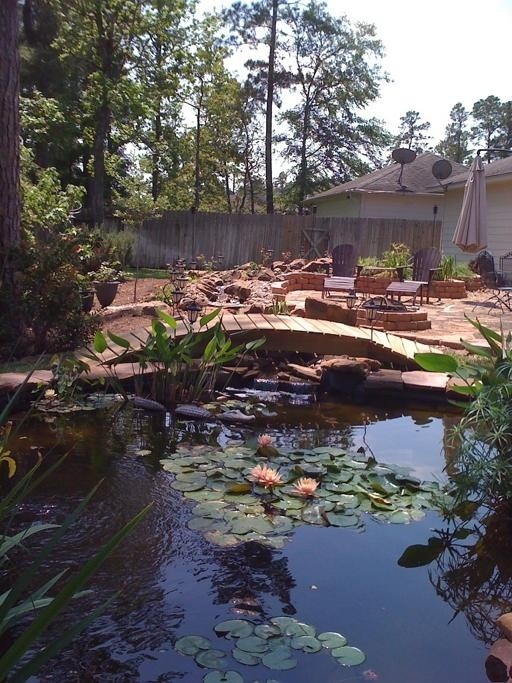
[385,281,422,306]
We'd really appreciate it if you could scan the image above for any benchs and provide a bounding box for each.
[320,277,358,299]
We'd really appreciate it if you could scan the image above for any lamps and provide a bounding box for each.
[170,253,224,286]
[185,298,202,325]
[172,287,185,304]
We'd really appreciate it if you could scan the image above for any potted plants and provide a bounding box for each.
[92,259,122,305]
[75,272,98,310]
